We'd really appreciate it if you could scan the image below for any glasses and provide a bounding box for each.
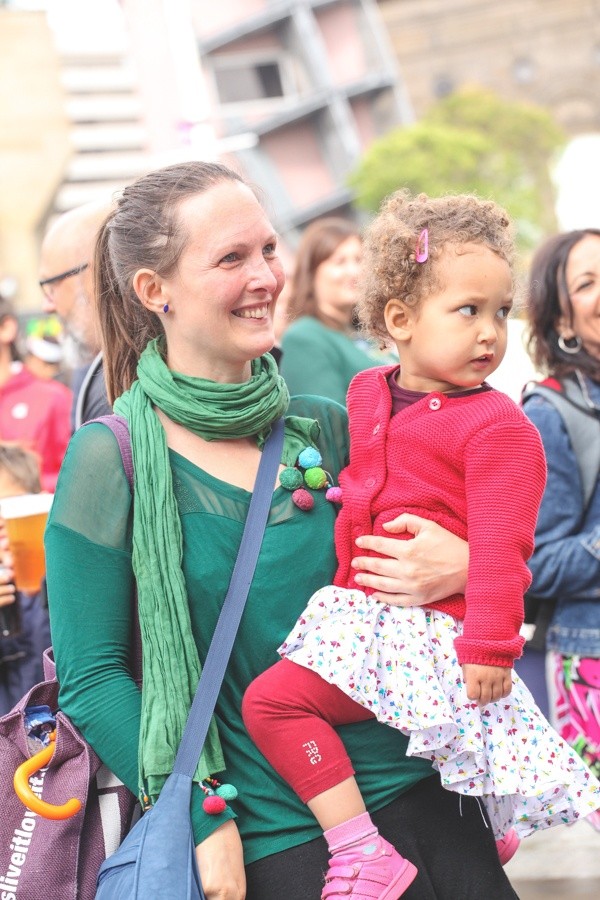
[40,264,88,301]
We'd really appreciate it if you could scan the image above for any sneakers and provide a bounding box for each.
[321,835,418,900]
[495,828,519,865]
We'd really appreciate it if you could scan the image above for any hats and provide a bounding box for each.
[26,335,62,362]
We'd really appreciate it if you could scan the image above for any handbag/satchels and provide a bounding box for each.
[0,646,136,900]
[94,773,206,900]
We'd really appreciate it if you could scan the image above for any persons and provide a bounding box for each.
[280,216,400,414]
[0,296,74,492]
[0,441,62,719]
[517,226,600,774]
[43,161,517,900]
[236,193,600,900]
[41,202,126,439]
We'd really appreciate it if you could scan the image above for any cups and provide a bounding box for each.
[0,494,55,591]
